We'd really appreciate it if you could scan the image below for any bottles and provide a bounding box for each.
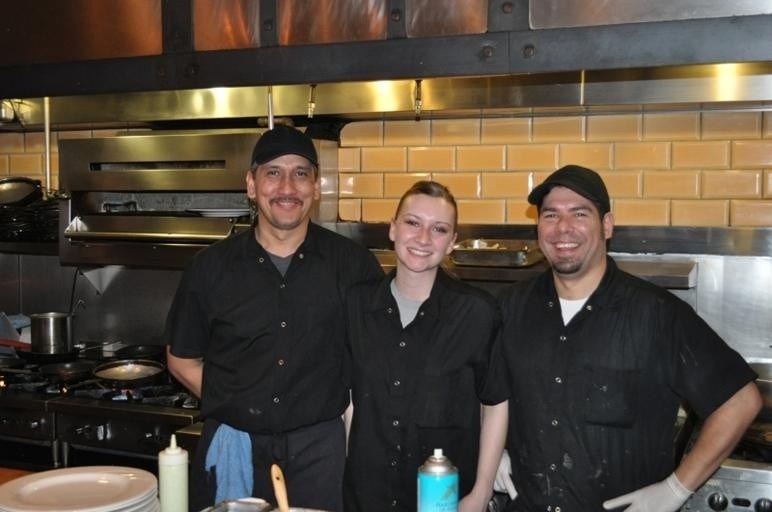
[416,447,460,512]
[157,433,189,512]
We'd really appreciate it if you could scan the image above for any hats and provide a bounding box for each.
[250,124,319,169]
[526,163,610,211]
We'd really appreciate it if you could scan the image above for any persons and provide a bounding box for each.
[342,177,513,511]
[164,127,388,512]
[495,163,765,512]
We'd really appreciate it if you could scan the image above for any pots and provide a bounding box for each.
[30,313,71,350]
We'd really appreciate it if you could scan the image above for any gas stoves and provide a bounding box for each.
[7,339,228,468]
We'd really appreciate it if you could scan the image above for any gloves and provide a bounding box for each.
[493,448,519,501]
[602,471,694,512]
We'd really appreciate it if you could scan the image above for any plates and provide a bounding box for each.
[0,465,157,512]
[192,205,248,219]
